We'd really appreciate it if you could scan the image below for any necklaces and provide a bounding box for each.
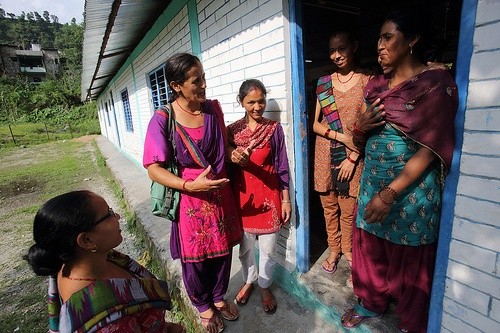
[176,98,201,116]
[66,275,96,281]
[337,66,358,84]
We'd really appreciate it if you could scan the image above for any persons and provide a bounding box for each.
[26,190,185,333]
[144,16,459,333]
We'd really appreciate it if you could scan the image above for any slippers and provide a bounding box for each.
[340,308,383,330]
[200,309,224,333]
[261,290,277,315]
[322,256,341,274]
[234,285,254,307]
[214,300,239,321]
[343,254,353,270]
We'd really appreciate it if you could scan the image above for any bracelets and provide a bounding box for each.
[325,129,330,138]
[347,157,356,164]
[383,186,399,200]
[282,200,291,203]
[333,130,338,140]
[378,189,393,204]
[182,178,194,191]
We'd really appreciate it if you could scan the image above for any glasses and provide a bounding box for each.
[85,208,116,233]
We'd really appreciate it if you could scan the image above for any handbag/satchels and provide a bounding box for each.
[150,159,180,221]
